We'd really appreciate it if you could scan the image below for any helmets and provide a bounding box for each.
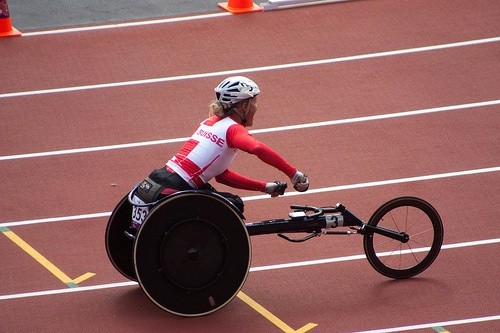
[213,76,260,109]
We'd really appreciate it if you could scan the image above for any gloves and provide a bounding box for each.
[265,182,285,197]
[291,171,309,192]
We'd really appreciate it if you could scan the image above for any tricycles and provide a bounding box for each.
[106,174,444,316]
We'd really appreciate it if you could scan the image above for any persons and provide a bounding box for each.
[136,75,310,215]
